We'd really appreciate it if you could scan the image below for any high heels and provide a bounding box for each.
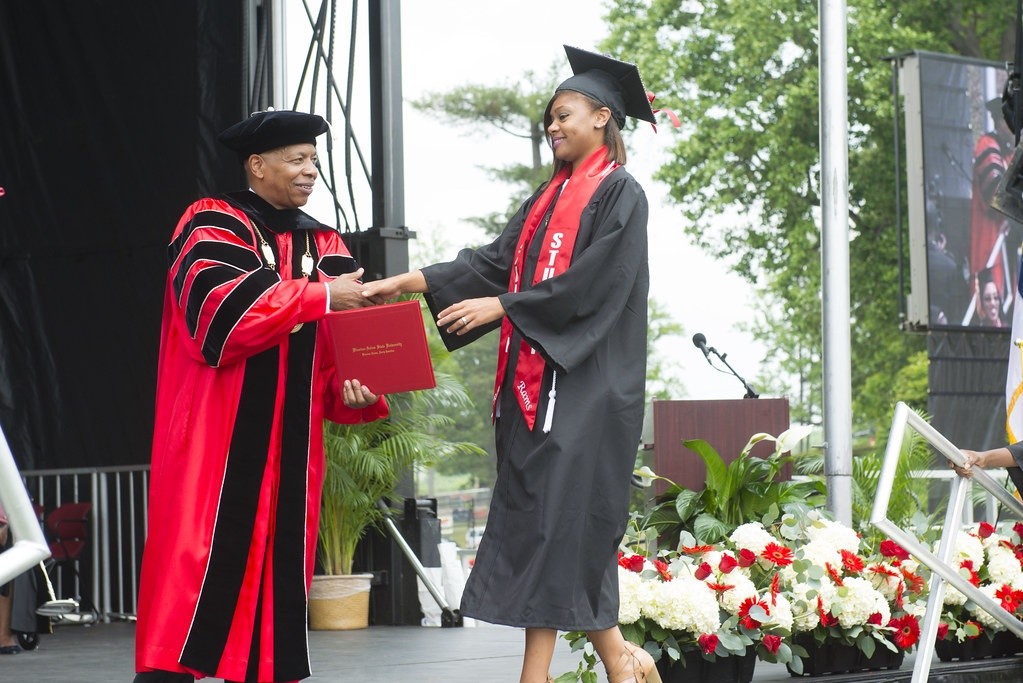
[607,641,663,683]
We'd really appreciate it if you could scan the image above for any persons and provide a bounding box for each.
[969,96,1022,319]
[361,43,665,683]
[133,109,391,683]
[0,504,21,655]
[948,440,1023,500]
[972,267,1009,327]
[926,198,980,327]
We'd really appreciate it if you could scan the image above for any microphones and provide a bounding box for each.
[692,332,711,363]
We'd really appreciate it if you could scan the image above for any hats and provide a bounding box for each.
[555,44,657,131]
[986,97,1004,118]
[217,111,330,164]
[978,269,992,289]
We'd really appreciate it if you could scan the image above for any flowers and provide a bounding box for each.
[554,404,1023,683]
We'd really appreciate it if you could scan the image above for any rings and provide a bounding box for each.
[462,317,467,325]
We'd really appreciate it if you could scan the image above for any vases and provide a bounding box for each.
[935,629,1023,663]
[642,642,756,683]
[785,631,905,678]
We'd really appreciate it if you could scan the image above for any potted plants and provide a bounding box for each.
[306,292,488,631]
